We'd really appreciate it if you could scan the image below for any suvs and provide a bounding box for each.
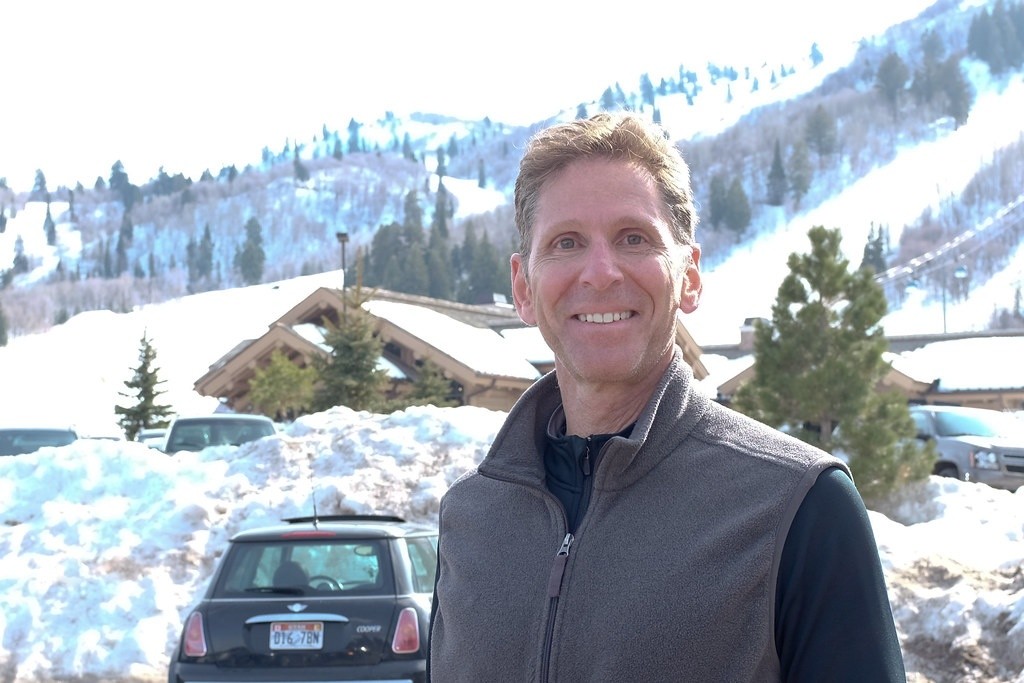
[168,514,439,683]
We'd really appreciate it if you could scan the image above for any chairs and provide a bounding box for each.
[274,561,311,586]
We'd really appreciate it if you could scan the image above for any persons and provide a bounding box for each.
[427,114,906,683]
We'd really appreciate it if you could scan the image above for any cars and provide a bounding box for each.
[0,414,282,457]
[829,404,1024,493]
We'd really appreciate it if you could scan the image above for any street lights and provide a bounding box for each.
[943,253,969,334]
